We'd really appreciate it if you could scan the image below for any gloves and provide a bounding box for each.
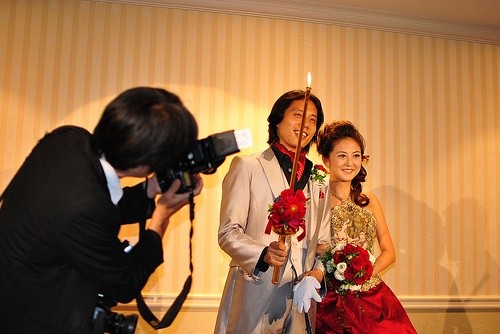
[292,276,322,314]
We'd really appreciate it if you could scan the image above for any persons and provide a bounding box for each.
[315,120,420,334]
[213,90,329,334]
[0,86,203,334]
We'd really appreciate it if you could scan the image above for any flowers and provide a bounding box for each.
[309,164,330,187]
[267,188,310,224]
[321,242,383,295]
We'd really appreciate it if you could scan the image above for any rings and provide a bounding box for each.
[268,255,273,259]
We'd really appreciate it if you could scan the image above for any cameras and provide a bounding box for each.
[155,128,254,194]
[93,294,139,334]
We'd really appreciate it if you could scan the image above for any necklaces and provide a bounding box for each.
[331,191,354,204]
